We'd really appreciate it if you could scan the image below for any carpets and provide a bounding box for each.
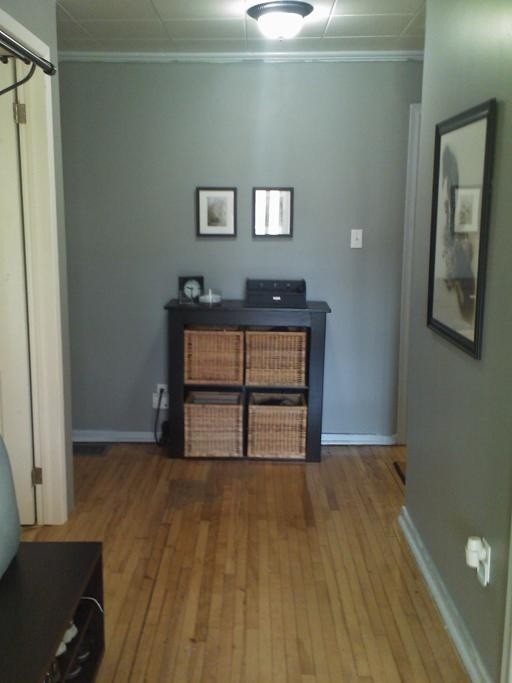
[393,459,407,483]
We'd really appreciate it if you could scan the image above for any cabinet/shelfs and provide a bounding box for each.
[162,298,332,463]
[0,541,106,683]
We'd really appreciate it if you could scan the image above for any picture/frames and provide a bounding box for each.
[450,185,482,236]
[252,186,295,238]
[427,97,498,362]
[194,185,237,238]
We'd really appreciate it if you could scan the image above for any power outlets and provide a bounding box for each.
[154,383,168,408]
[475,536,493,587]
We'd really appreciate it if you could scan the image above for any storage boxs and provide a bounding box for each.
[182,326,309,460]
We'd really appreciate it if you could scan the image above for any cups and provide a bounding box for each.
[178,290,192,306]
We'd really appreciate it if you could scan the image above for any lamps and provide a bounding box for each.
[246,0,315,40]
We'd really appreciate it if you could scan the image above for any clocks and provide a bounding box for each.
[181,279,202,301]
